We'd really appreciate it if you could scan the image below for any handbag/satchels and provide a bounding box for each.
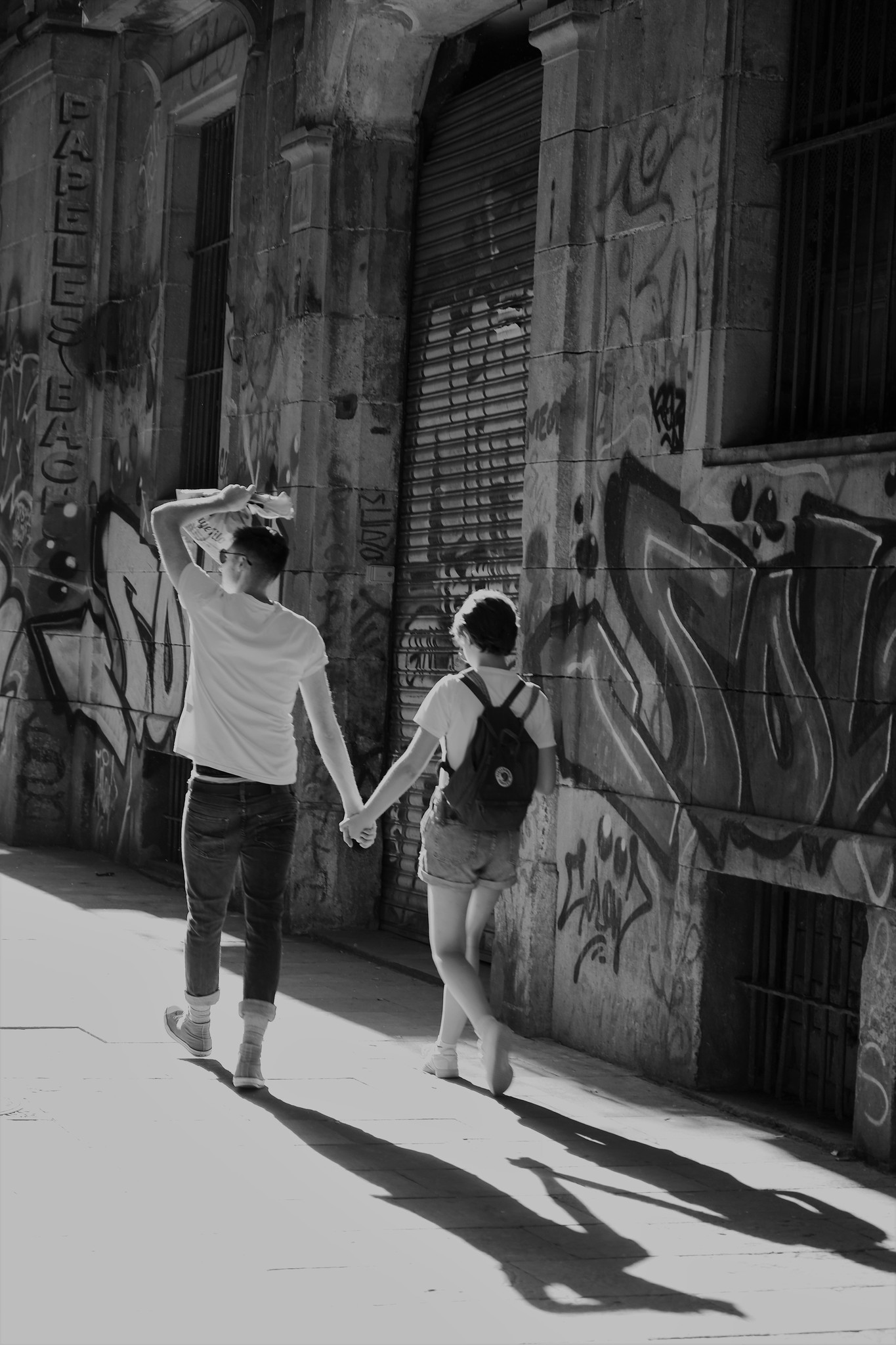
[175,485,295,565]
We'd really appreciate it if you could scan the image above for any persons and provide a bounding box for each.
[336,588,558,1095]
[151,483,375,1089]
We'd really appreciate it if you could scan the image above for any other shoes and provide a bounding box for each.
[422,1048,460,1078]
[478,1020,514,1096]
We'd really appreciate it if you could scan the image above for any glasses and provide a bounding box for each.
[219,548,253,566]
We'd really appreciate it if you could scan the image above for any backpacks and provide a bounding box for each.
[435,667,541,833]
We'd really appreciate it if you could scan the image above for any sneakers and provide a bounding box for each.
[163,1005,213,1057]
[232,1043,266,1089]
